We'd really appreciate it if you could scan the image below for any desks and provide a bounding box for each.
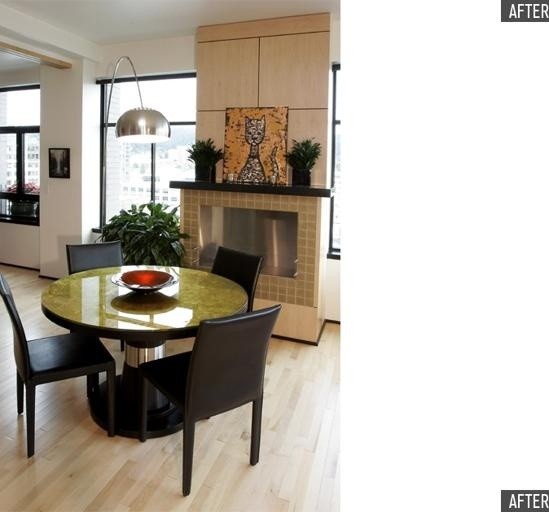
[40,263,250,444]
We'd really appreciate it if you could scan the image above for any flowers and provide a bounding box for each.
[6,182,40,200]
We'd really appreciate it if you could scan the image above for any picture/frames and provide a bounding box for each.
[48,147,72,179]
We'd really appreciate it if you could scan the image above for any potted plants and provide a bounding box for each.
[282,132,322,186]
[185,137,223,183]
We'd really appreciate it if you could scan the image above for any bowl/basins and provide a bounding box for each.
[111,270,177,293]
[111,290,179,315]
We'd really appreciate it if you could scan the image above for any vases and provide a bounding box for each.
[11,201,36,217]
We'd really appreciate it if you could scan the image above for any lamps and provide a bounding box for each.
[97,55,174,243]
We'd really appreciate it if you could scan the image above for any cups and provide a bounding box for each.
[227,174,234,182]
[269,176,277,185]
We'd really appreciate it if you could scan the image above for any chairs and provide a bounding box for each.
[0,278,117,460]
[207,245,265,310]
[65,237,128,354]
[136,303,284,497]
